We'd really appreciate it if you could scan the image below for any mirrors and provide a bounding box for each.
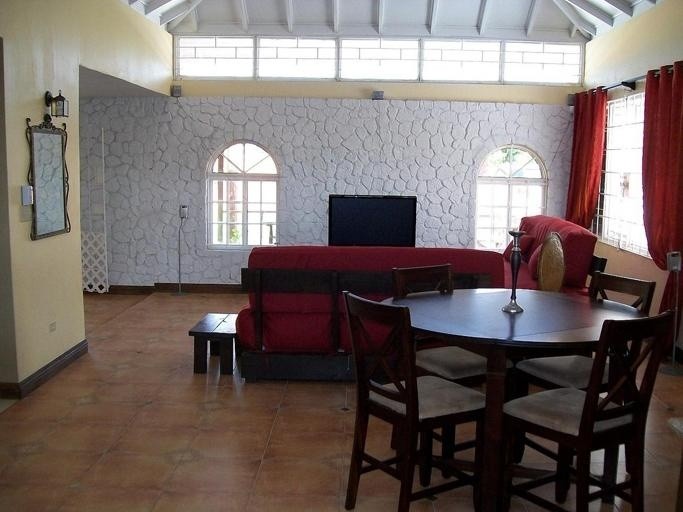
[26,114,71,241]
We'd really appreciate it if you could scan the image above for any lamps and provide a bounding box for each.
[171,204,190,295]
[660,252,683,376]
[46,90,69,117]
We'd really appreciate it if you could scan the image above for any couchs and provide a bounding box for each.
[503,215,607,296]
[236,246,505,382]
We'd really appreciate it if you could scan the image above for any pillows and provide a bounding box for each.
[503,235,536,262]
[528,243,542,281]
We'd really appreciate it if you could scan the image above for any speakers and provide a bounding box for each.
[173,85,181,97]
[567,93,574,106]
[371,91,384,100]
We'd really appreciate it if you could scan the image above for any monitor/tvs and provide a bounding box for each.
[329,193,417,247]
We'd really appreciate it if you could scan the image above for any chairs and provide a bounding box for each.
[513,271,658,471]
[393,265,489,479]
[496,310,671,511]
[343,290,487,511]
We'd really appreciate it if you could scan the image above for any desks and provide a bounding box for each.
[379,289,651,511]
